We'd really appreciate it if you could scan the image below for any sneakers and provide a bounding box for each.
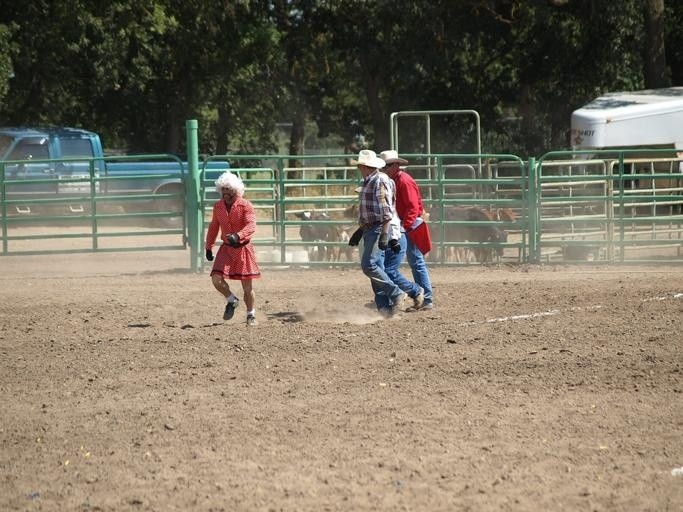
[406,287,433,312]
[392,291,408,315]
[247,315,257,326]
[223,296,240,320]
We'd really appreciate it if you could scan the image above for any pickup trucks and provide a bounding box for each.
[1,126,230,229]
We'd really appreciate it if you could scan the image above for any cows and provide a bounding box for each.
[293,201,360,269]
[425,206,516,265]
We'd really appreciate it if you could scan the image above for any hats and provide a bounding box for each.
[350,150,408,169]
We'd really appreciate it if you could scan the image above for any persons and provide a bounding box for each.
[346,148,425,317]
[376,150,434,311]
[204,172,261,327]
[381,178,426,313]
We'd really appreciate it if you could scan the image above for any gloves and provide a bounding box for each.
[348,229,363,246]
[378,233,388,250]
[205,250,214,261]
[388,239,401,255]
[225,233,238,245]
